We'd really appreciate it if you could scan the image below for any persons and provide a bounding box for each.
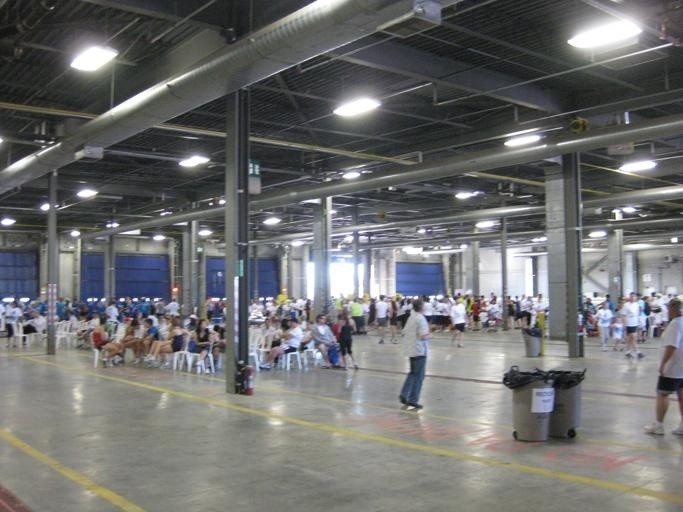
[1,291,682,439]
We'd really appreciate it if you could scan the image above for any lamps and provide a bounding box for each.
[69,44,121,73]
[333,94,382,119]
[566,17,644,51]
[178,154,211,168]
[618,159,658,173]
[76,188,99,199]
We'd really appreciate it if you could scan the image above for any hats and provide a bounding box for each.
[287,318,298,323]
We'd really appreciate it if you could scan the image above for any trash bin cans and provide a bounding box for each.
[522,327,542,358]
[509,370,583,441]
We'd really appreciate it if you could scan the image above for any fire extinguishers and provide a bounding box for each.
[245,364,255,396]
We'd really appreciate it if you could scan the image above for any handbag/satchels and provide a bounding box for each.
[328,344,338,363]
[187,341,198,352]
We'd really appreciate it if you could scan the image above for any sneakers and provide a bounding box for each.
[5,342,16,348]
[259,363,271,369]
[644,421,664,435]
[195,359,210,374]
[322,364,362,371]
[133,357,170,369]
[399,394,423,408]
[672,422,682,436]
[376,327,499,348]
[600,346,646,359]
